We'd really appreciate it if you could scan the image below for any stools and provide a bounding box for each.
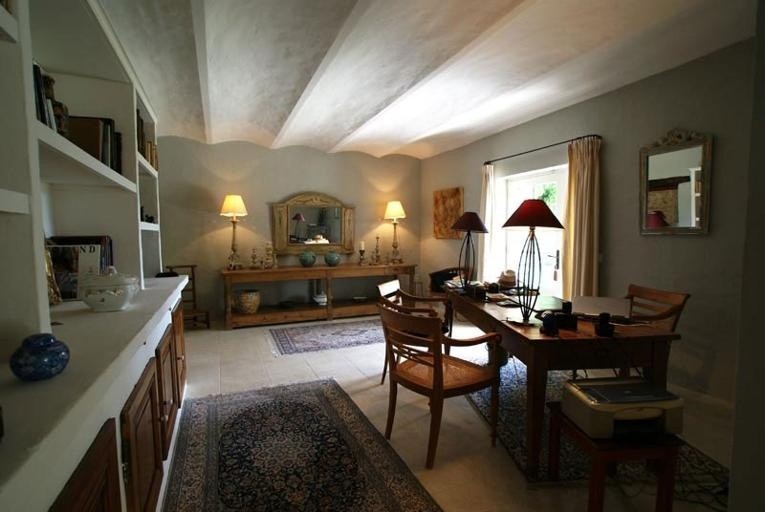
[545,398,681,511]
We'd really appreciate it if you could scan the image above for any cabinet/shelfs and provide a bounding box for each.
[688,167,702,227]
[0,1,188,512]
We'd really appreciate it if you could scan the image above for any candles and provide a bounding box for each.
[359,239,366,252]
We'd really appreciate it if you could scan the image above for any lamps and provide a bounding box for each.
[502,199,566,331]
[647,210,670,228]
[291,211,305,241]
[218,195,248,270]
[383,201,406,264]
[451,213,488,292]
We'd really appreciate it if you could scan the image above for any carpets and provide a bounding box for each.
[263,318,387,356]
[464,355,730,492]
[161,378,442,511]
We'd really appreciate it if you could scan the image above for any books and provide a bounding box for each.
[33,63,122,174]
[46,236,113,301]
[137,109,159,171]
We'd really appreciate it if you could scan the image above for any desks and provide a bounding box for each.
[218,263,417,331]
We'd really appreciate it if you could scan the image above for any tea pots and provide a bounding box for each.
[313,291,328,306]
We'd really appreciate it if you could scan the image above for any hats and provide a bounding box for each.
[498,270,524,288]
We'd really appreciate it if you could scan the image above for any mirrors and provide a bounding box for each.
[268,192,355,254]
[638,128,714,235]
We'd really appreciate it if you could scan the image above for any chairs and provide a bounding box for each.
[166,262,210,331]
[377,277,452,384]
[429,268,471,310]
[574,285,690,382]
[378,305,502,471]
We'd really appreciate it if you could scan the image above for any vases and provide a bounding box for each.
[231,289,261,317]
[299,249,315,267]
[323,250,343,266]
